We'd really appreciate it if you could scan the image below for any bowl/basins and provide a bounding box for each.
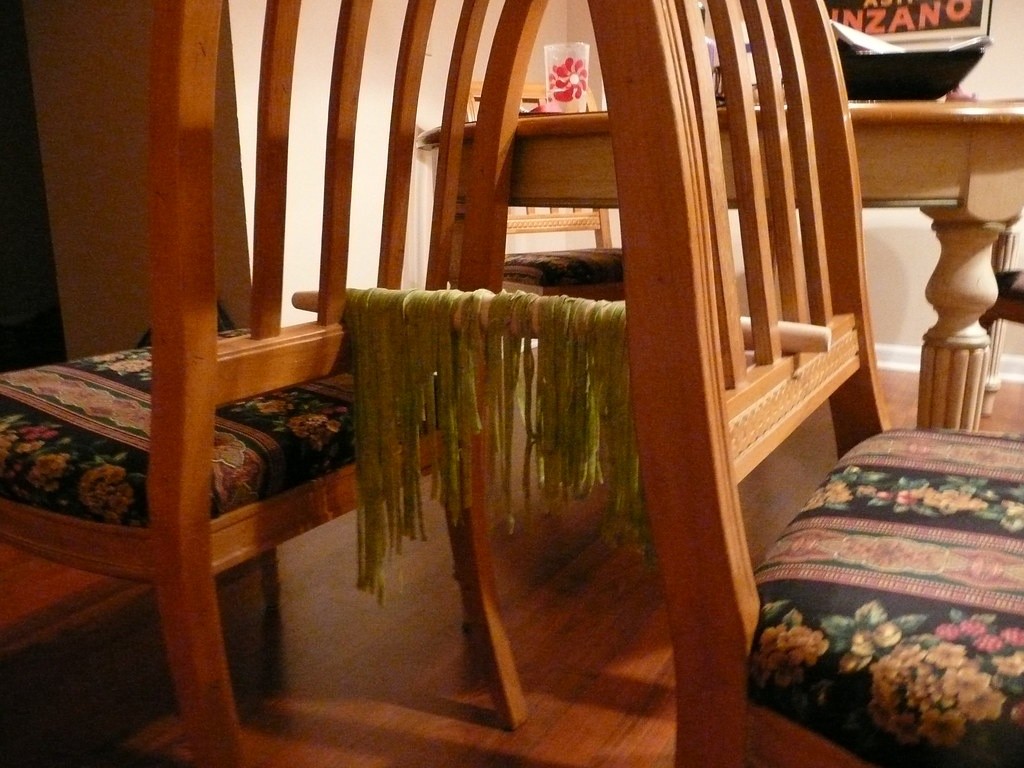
[836,49,986,103]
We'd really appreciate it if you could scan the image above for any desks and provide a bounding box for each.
[412,98,1024,434]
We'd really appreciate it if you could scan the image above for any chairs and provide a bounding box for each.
[0,0,549,768]
[464,79,626,302]
[588,0,1024,767]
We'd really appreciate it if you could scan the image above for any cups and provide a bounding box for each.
[544,43,590,114]
